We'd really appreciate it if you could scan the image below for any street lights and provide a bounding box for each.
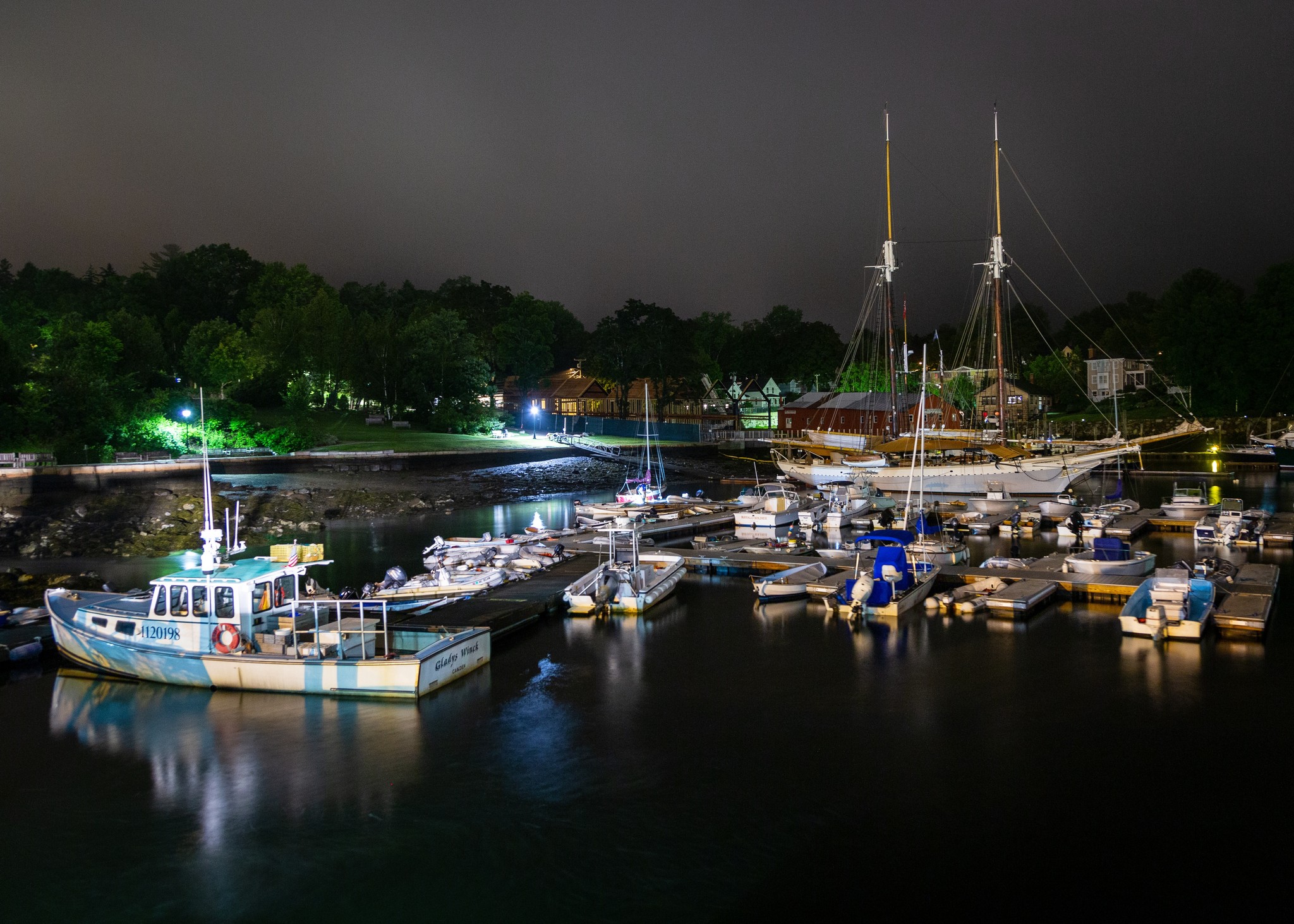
[530,407,537,439]
[182,409,191,448]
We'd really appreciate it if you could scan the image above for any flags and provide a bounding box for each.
[281,539,298,568]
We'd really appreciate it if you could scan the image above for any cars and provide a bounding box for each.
[984,415,1007,424]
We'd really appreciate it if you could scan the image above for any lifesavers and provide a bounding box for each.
[211,622,239,653]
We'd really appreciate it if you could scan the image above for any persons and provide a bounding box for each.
[275,579,285,606]
[639,481,649,504]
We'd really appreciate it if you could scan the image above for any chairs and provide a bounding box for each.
[708,536,717,541]
[1150,590,1185,620]
[881,565,903,599]
[721,536,732,541]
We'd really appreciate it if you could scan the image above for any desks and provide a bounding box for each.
[309,617,380,656]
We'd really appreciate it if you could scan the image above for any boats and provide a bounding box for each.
[1203,354,1294,471]
[748,561,828,598]
[43,387,492,701]
[1118,568,1217,641]
[563,516,687,614]
[357,459,1294,617]
[925,576,1010,613]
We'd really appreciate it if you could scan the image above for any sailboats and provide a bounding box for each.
[614,379,667,505]
[771,100,1215,498]
[806,343,941,617]
